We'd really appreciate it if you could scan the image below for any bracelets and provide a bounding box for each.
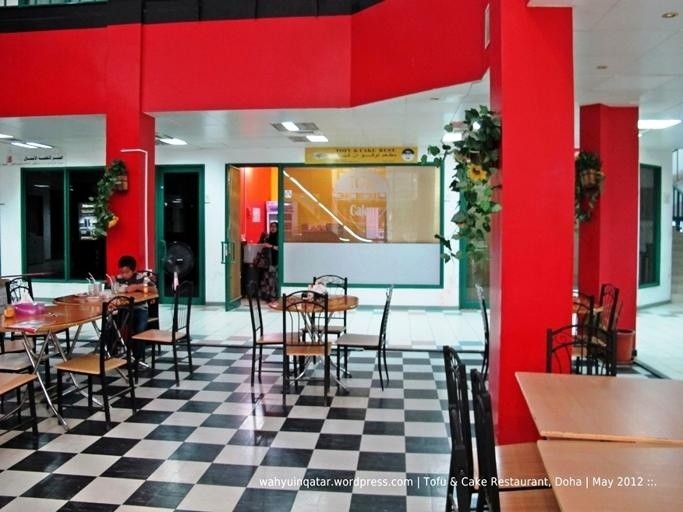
[271,245,274,249]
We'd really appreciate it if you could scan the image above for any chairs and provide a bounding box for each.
[245,274,392,398]
[442,346,559,511]
[475,284,492,378]
[547,283,620,375]
[473,370,554,488]
[0,271,192,449]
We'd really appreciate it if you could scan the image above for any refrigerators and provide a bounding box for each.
[265,200,298,236]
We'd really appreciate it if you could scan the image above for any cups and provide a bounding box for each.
[110,281,118,294]
[96,284,104,294]
[86,283,97,296]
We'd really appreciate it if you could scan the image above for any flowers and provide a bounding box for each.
[421,105,502,263]
[92,159,127,238]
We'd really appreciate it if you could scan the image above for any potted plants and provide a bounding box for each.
[575,151,604,223]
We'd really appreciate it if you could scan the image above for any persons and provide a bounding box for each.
[263,221,278,298]
[99,255,155,371]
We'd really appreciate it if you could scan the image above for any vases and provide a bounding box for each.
[116,176,128,190]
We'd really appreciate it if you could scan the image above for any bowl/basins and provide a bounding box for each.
[117,286,127,292]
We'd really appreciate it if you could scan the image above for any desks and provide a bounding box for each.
[515,372,683,441]
[538,439,683,511]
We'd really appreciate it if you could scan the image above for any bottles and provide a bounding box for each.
[142,276,148,295]
